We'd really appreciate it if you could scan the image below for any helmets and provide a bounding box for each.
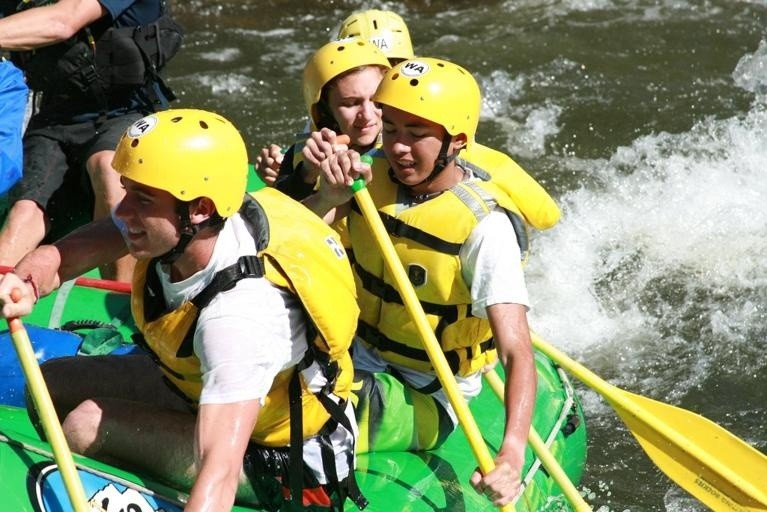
[110,108,250,266]
[336,8,414,60]
[301,37,393,155]
[369,56,482,187]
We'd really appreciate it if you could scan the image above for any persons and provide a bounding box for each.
[273,36,394,200]
[299,58,539,507]
[0,108,358,512]
[1,0,182,280]
[254,9,413,185]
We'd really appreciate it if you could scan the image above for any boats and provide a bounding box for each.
[1,160,587,510]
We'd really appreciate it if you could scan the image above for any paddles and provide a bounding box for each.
[530,329,763,512]
[481,366,596,512]
[0,296,94,512]
[0,263,133,293]
[340,155,521,512]
